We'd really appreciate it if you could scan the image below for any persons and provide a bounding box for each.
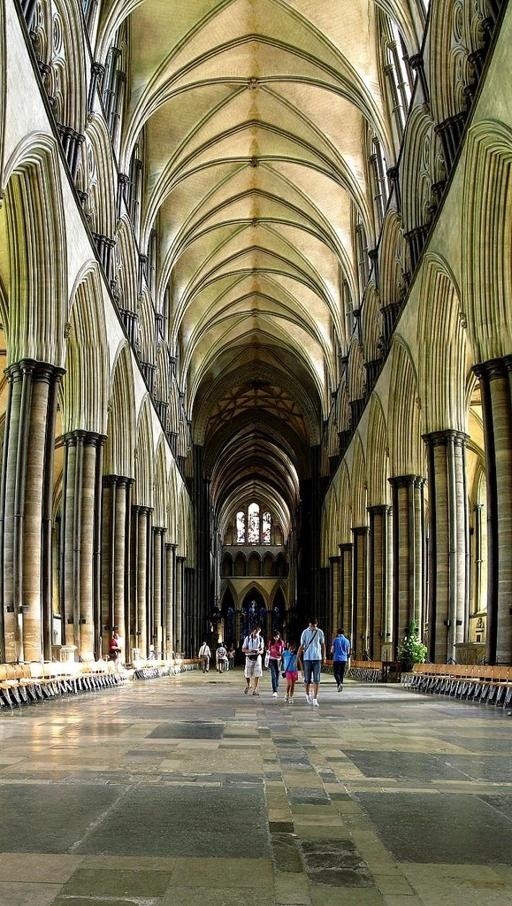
[242,626,265,696]
[265,631,286,697]
[281,641,304,704]
[215,639,239,673]
[109,632,121,662]
[198,641,211,673]
[300,617,327,707]
[331,628,350,692]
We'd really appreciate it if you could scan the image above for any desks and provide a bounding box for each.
[382,660,402,682]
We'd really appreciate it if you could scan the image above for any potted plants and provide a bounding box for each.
[397,617,428,672]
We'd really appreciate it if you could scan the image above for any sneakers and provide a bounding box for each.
[306,692,319,706]
[245,687,259,695]
[338,683,343,691]
[285,695,293,704]
[273,692,277,697]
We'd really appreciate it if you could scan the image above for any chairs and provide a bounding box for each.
[0,658,201,709]
[400,663,512,710]
[321,659,382,683]
